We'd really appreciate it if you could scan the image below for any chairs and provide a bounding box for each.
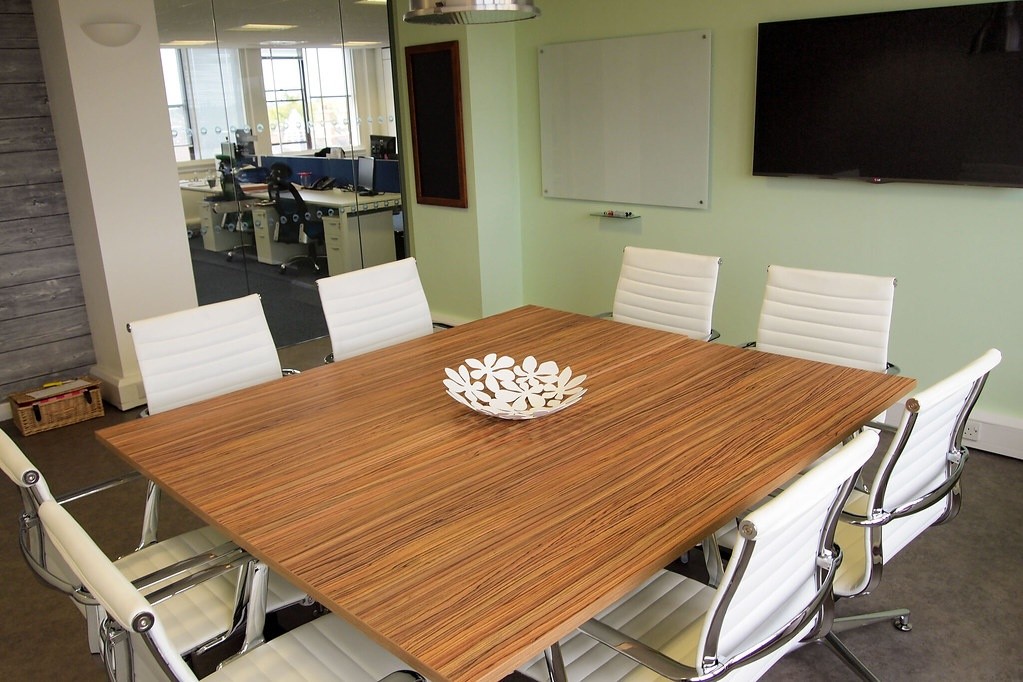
[211,173,258,262]
[591,245,901,547]
[0,426,436,681]
[513,349,1003,681]
[268,182,327,272]
[316,257,441,364]
[126,293,301,419]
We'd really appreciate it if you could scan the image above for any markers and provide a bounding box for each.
[603,209,633,216]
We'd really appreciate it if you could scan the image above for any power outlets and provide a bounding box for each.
[962,421,981,440]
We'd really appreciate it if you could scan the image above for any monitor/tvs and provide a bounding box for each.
[222,141,236,162]
[753,0,1023,189]
[357,156,378,196]
[370,135,396,160]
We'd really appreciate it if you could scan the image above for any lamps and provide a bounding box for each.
[403,0,540,25]
[81,23,141,47]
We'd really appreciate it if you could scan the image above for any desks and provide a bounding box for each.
[94,305,916,682]
[180,179,401,277]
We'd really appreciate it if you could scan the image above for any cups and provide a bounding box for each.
[298,172,312,187]
[207,180,216,188]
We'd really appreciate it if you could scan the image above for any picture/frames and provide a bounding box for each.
[405,40,468,209]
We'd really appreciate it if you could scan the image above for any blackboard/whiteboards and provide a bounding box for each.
[404,39,470,209]
[537,27,711,208]
[752,0,1023,190]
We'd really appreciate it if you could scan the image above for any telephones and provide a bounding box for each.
[311,176,336,190]
